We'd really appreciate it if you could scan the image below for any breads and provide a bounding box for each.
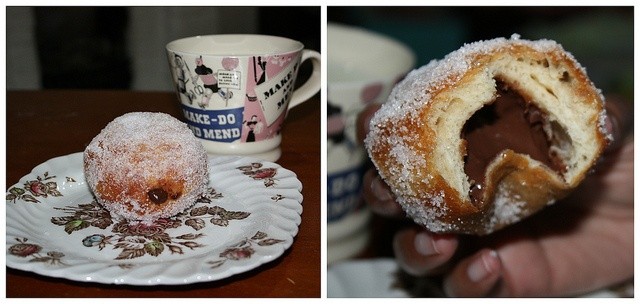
[81,111,209,221]
[364,32,614,239]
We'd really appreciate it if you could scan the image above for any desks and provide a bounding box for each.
[7,86,321,295]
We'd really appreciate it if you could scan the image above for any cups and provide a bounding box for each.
[164,33,321,164]
[327,22,416,266]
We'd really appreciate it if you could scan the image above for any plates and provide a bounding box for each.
[6,149,303,287]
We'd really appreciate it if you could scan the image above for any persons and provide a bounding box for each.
[355,91,634,297]
